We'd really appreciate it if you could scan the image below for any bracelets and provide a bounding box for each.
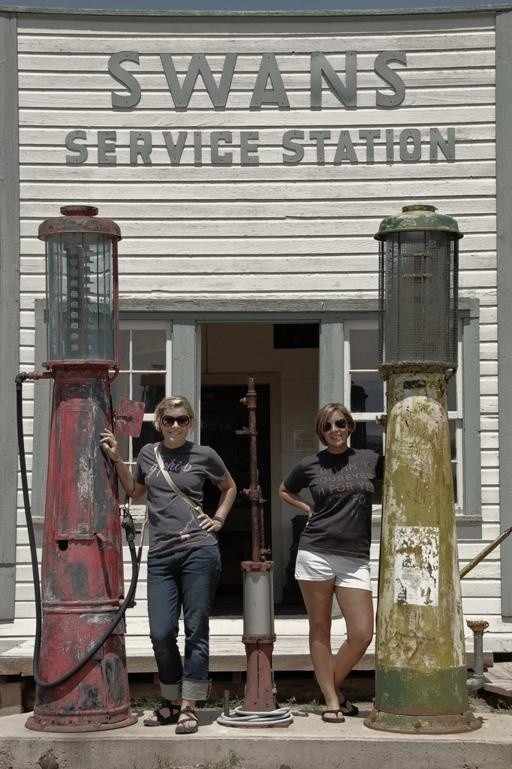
[113,456,122,463]
[213,514,224,524]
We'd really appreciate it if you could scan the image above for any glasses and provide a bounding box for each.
[323,419,346,431]
[162,414,190,427]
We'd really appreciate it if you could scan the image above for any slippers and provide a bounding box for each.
[338,697,359,716]
[322,708,345,723]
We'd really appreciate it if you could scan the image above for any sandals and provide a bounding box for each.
[175,707,199,733]
[144,703,180,726]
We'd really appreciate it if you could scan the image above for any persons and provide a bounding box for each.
[279,405,388,722]
[98,393,239,732]
